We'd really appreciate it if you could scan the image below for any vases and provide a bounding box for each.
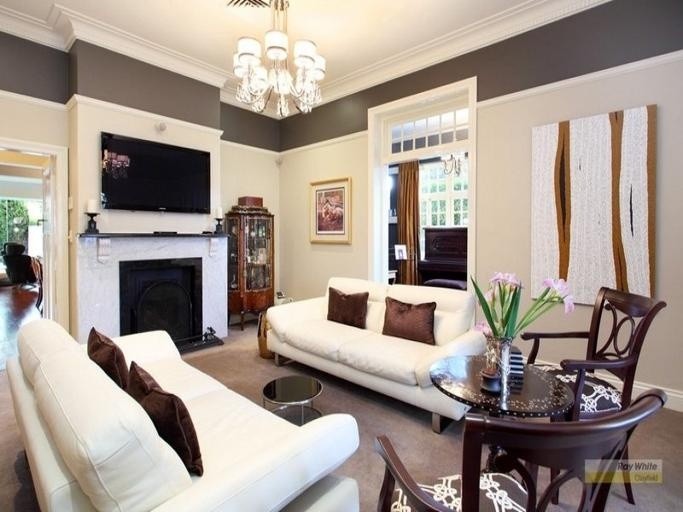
[482,333,514,389]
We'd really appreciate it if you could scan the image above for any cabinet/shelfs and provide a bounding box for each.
[226,196,275,331]
[412,226,468,286]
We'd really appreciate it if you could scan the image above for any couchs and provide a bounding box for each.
[6,318,360,511]
[265,276,492,435]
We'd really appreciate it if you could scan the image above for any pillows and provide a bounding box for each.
[379,284,476,345]
[323,277,385,331]
[16,318,76,381]
[89,327,128,389]
[32,344,194,512]
[129,359,204,477]
[382,297,436,346]
[325,286,369,329]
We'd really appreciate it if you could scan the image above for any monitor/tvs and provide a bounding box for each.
[100,130,210,215]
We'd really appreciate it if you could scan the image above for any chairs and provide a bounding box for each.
[372,389,669,511]
[517,287,667,505]
[0,242,43,308]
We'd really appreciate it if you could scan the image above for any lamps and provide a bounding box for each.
[232,0,330,118]
[438,152,469,175]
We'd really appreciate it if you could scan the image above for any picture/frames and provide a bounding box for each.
[309,176,352,244]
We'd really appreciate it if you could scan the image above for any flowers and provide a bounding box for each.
[467,269,577,333]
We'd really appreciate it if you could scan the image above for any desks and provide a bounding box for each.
[262,375,323,424]
